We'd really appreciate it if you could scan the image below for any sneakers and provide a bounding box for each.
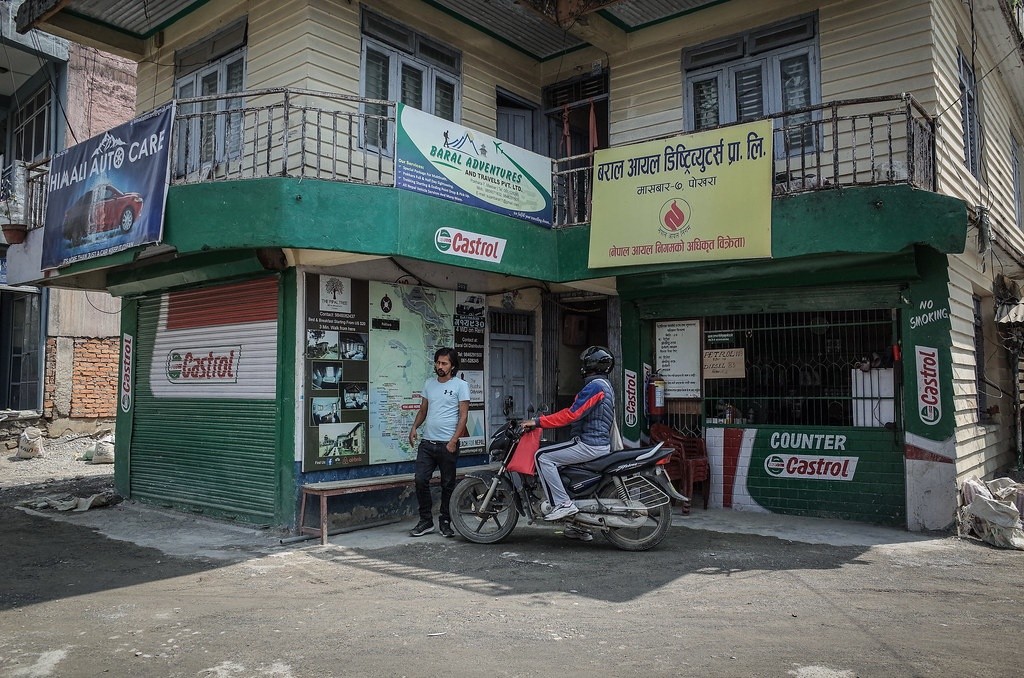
[544,502,579,521]
[410,519,435,536]
[563,528,593,541]
[438,520,455,537]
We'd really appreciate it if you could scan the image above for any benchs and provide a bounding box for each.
[299,462,506,545]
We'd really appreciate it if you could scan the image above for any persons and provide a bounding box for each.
[409,348,470,539]
[521,345,615,520]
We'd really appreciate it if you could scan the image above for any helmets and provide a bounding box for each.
[580,346,614,374]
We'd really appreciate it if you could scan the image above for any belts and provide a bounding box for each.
[426,440,449,444]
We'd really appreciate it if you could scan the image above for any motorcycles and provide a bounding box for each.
[449,394,693,552]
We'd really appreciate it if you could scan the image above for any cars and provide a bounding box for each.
[62,183,144,248]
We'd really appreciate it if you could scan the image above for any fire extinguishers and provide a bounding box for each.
[648,368,665,416]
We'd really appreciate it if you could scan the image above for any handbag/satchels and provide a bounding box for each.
[608,419,624,452]
[507,427,543,476]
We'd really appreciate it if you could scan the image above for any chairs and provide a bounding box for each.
[648,424,711,516]
